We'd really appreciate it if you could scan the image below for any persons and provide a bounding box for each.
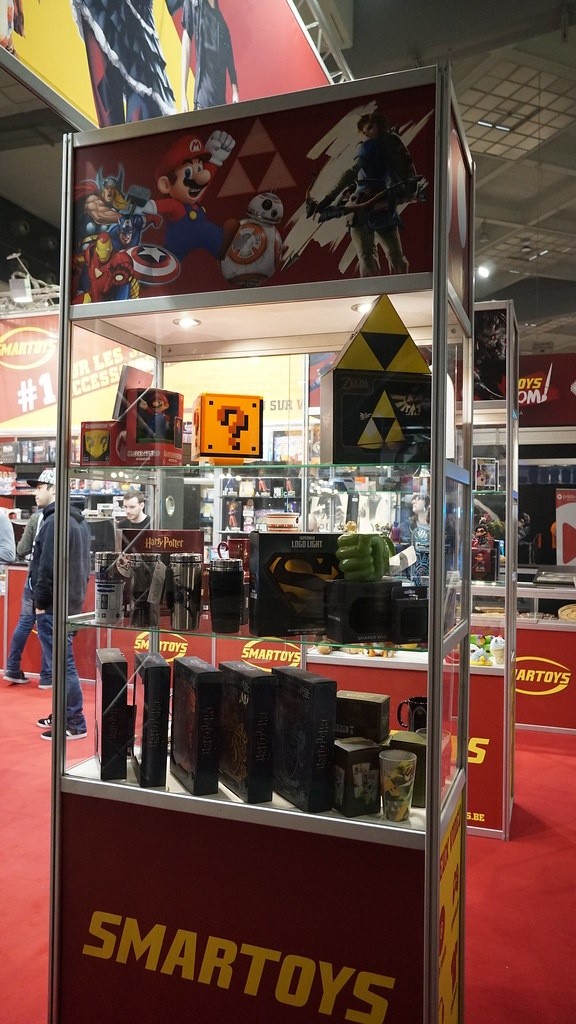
[551,522,556,564]
[0,470,89,741]
[475,525,489,548]
[391,494,430,544]
[116,490,150,529]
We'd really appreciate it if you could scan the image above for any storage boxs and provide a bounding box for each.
[217,660,278,804]
[334,683,391,743]
[171,655,226,795]
[272,665,338,814]
[117,527,205,618]
[334,736,383,818]
[247,531,345,637]
[389,730,428,809]
[0,440,56,464]
[92,648,129,782]
[135,651,171,788]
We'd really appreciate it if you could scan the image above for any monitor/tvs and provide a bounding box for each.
[85,516,116,553]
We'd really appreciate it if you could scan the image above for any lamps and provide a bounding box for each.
[8,272,34,304]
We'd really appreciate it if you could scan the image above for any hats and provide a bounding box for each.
[27,470,56,488]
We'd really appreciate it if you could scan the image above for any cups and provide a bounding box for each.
[379,750,417,822]
[397,696,427,732]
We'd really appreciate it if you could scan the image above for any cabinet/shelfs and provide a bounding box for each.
[49,59,476,1024]
[184,462,428,1024]
[297,300,519,841]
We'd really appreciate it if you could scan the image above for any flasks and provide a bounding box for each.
[94,550,126,628]
[128,552,163,628]
[169,552,203,631]
[209,558,245,633]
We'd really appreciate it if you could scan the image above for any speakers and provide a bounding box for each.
[161,477,199,530]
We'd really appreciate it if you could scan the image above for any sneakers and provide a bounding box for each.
[3,670,30,683]
[37,714,52,728]
[41,726,87,741]
[38,679,53,689]
